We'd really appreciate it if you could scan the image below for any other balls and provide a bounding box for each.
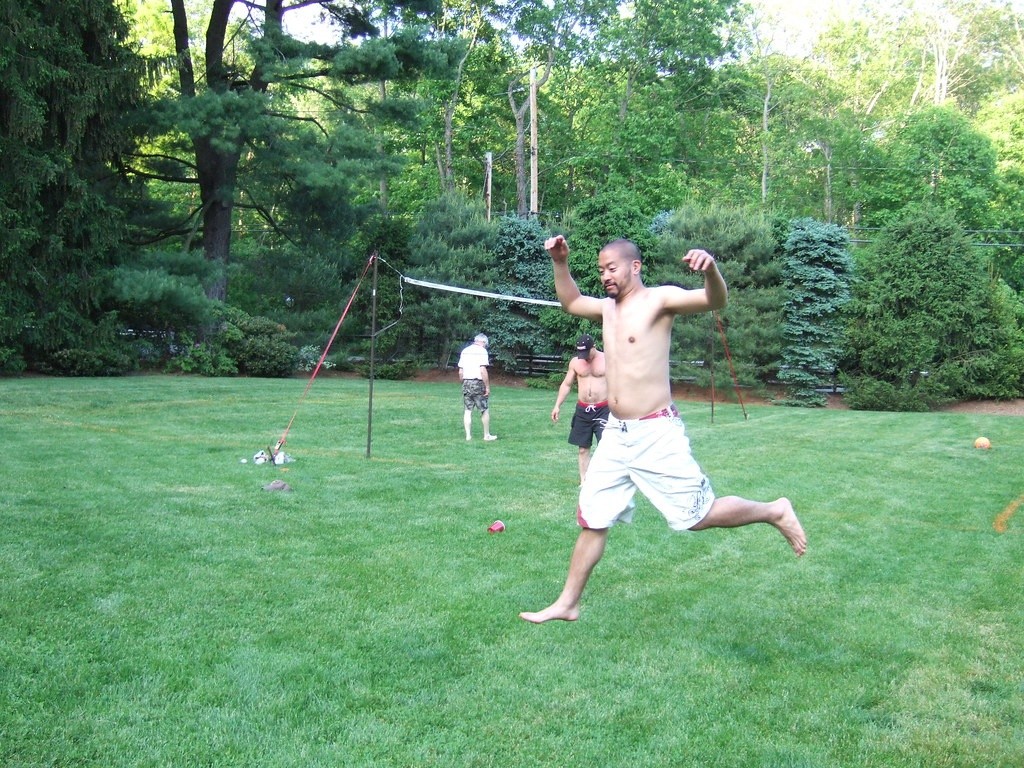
[975,437,991,450]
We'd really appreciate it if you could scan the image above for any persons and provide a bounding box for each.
[457,333,497,440]
[550,335,611,487]
[520,236,808,622]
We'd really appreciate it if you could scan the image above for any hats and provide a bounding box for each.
[576,334,594,359]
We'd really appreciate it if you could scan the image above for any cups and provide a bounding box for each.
[487,520,506,534]
